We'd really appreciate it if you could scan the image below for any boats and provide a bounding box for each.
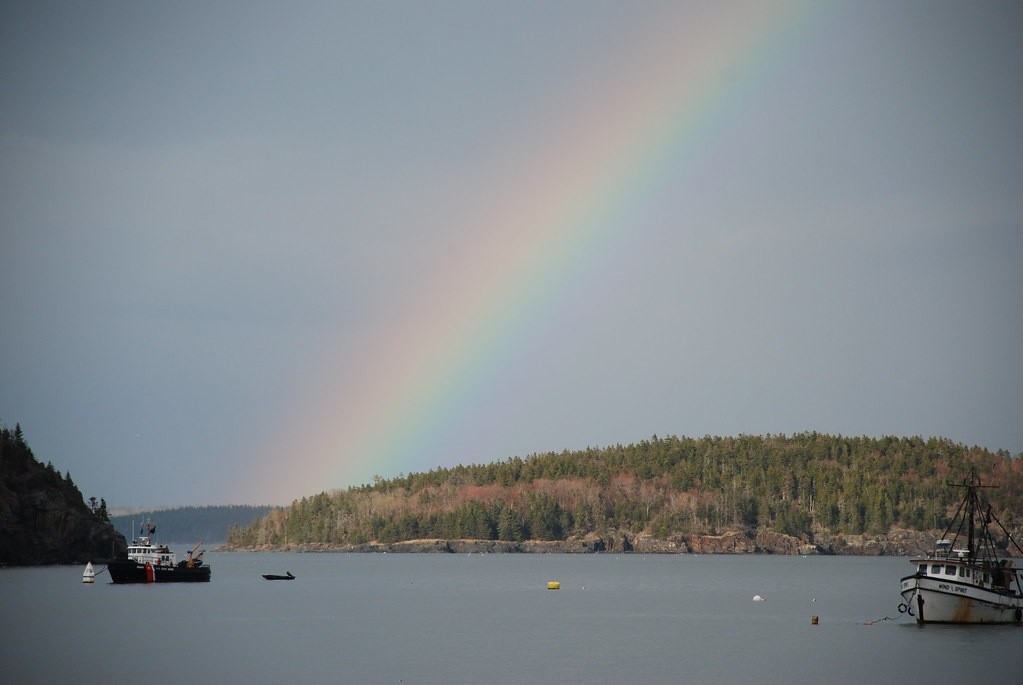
[899,463,1023,625]
[107,514,213,583]
[261,571,297,580]
